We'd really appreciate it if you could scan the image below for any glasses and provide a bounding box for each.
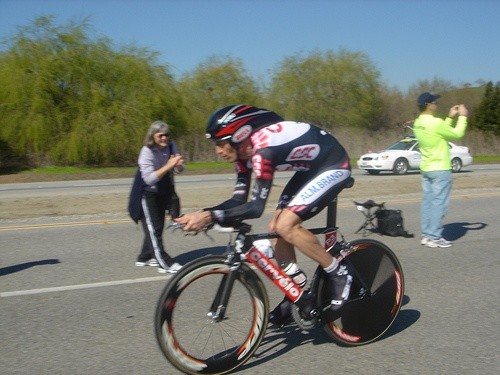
[152,133,168,138]
[426,102,438,104]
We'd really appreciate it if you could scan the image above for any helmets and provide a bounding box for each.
[204,105,287,147]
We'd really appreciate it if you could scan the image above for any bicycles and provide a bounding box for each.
[153,176,405,375]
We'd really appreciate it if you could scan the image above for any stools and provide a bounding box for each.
[354,199,384,239]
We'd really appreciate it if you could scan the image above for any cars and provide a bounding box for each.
[356,135,474,176]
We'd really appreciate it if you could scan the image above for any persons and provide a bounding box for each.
[414,91,469,247]
[175,103,351,323]
[127,121,184,273]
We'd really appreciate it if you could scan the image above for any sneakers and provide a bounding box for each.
[427,238,454,248]
[330,263,356,310]
[158,262,183,274]
[136,258,159,267]
[268,289,311,322]
[421,237,430,244]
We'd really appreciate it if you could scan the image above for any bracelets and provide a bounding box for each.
[447,115,454,119]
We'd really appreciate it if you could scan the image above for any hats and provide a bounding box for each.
[417,92,441,105]
